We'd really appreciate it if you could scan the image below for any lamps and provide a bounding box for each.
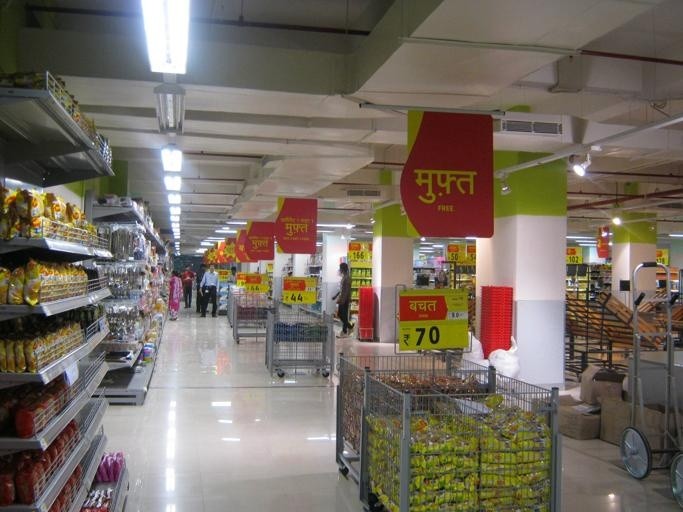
[501,178,512,196]
[573,153,593,177]
[140,1,192,221]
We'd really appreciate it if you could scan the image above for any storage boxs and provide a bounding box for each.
[532,365,683,461]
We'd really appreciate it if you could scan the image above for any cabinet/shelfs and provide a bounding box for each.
[334,356,561,512]
[223,283,279,341]
[0,68,174,512]
[265,311,333,379]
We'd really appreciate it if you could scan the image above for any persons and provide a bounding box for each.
[195,264,209,313]
[331,262,354,338]
[226,266,236,283]
[180,264,194,308]
[198,264,219,317]
[433,271,448,289]
[164,270,183,321]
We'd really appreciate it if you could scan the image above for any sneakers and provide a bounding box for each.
[337,325,355,338]
